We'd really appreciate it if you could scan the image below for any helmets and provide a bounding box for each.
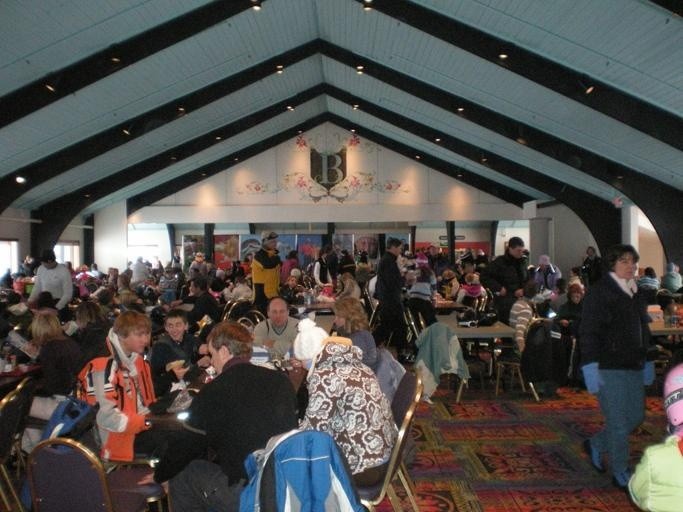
[479,310,498,325]
[465,272,480,285]
[456,309,478,328]
[664,363,683,426]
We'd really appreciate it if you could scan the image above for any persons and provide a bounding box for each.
[580,244,657,490]
[0,229,683,512]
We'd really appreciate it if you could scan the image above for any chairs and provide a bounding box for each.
[4,295,427,511]
[428,291,683,406]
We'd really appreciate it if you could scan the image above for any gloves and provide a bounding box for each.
[582,362,604,393]
[642,361,656,386]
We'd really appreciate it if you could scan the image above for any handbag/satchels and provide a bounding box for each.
[21,394,69,454]
[148,389,197,414]
[152,304,175,325]
[388,329,414,349]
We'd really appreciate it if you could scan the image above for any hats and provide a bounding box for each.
[293,317,329,360]
[97,289,114,305]
[120,270,132,287]
[415,252,428,264]
[261,230,278,241]
[539,255,549,265]
[667,262,674,272]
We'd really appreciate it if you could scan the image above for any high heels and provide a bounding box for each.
[611,468,630,491]
[584,438,606,472]
